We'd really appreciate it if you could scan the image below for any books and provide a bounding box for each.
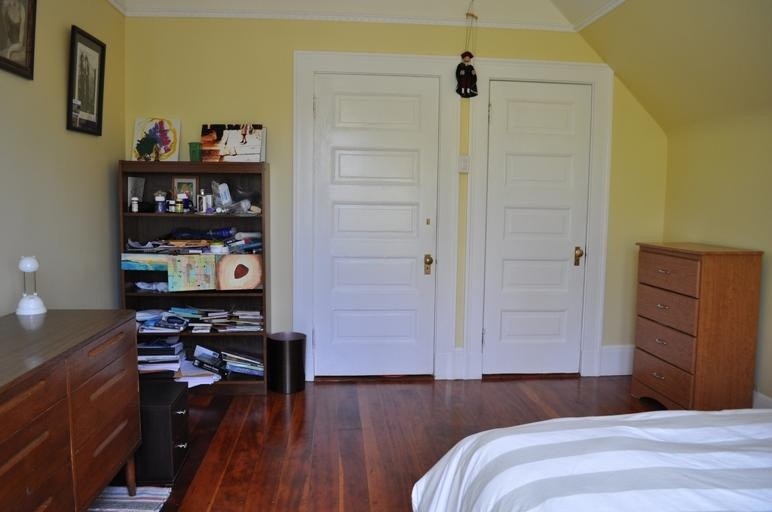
[125,223,263,254]
[133,306,265,388]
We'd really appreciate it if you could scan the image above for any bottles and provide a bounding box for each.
[130,196,140,213]
[154,192,166,213]
[169,199,176,213]
[196,187,206,213]
[176,193,186,214]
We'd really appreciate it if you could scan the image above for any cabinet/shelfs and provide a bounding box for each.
[0,352,77,512]
[65,315,142,507]
[117,160,270,390]
[109,382,192,485]
[623,240,765,412]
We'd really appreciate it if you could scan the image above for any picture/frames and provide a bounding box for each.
[171,175,199,212]
[0,0,37,82]
[66,24,107,137]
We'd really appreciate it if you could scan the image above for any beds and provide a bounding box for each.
[414,400,772,512]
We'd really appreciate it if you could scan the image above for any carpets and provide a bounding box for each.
[82,484,172,512]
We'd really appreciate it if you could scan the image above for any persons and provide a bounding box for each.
[455,51,478,98]
[238,125,252,145]
[0,0,26,59]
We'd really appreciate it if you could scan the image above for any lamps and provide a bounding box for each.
[15,255,47,315]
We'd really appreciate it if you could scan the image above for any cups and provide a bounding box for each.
[204,194,214,213]
[189,142,201,162]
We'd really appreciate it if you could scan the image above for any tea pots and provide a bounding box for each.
[181,198,194,214]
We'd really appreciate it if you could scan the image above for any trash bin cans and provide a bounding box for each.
[266,332,306,394]
